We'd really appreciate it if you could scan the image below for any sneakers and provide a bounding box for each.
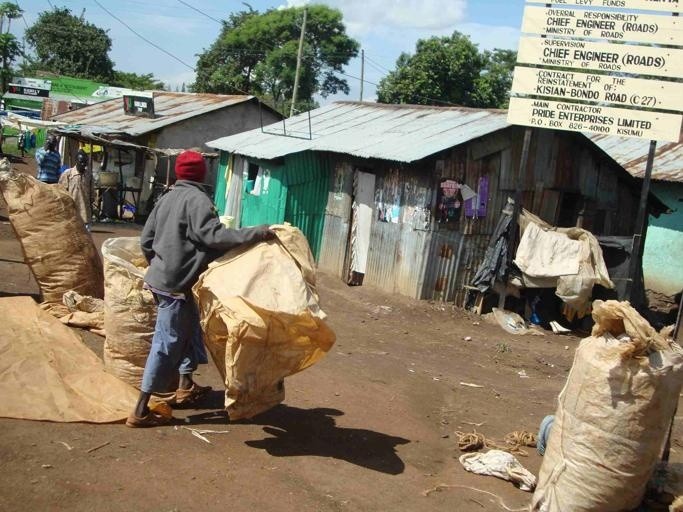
[175,382,212,403]
[126,411,168,428]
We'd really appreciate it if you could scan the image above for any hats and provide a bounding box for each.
[175,150,205,182]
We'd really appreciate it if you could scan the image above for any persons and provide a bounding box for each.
[17,130,28,158]
[35,134,61,185]
[57,149,97,235]
[123,150,276,429]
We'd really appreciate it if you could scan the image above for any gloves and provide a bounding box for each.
[262,224,276,242]
